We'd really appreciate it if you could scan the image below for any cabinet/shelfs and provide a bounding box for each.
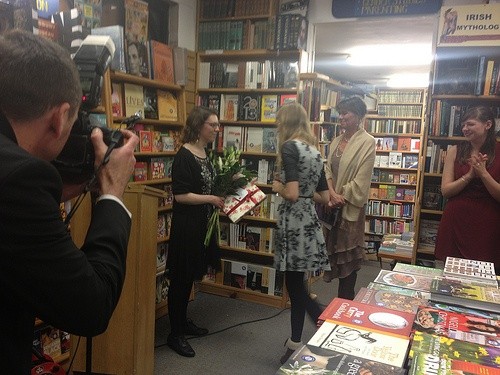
[86,0,500,309]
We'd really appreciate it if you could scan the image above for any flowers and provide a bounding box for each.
[202,140,259,248]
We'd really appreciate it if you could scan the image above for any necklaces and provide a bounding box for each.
[336,143,343,157]
[345,136,349,143]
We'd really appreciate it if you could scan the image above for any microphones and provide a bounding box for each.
[51,34,123,184]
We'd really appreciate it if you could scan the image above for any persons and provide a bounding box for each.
[450,288,478,298]
[433,106,500,276]
[272,102,332,365]
[127,42,147,77]
[163,105,248,357]
[298,18,307,50]
[449,281,477,290]
[423,308,500,334]
[0,28,140,375]
[315,95,374,300]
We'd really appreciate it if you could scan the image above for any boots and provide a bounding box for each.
[280,337,303,365]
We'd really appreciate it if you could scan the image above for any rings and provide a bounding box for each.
[476,163,480,167]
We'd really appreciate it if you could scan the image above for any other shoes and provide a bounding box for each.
[167,341,195,357]
[185,320,206,338]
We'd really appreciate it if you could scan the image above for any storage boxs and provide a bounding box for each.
[221,182,266,223]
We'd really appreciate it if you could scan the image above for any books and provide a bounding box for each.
[31,327,72,359]
[425,99,469,174]
[474,55,500,96]
[365,119,421,234]
[274,263,500,375]
[430,279,500,312]
[90,0,342,297]
[379,232,415,258]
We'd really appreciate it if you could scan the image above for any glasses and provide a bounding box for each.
[204,121,220,128]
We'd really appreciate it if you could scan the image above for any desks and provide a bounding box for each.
[376,233,413,265]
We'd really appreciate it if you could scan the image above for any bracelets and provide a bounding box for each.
[462,176,468,184]
